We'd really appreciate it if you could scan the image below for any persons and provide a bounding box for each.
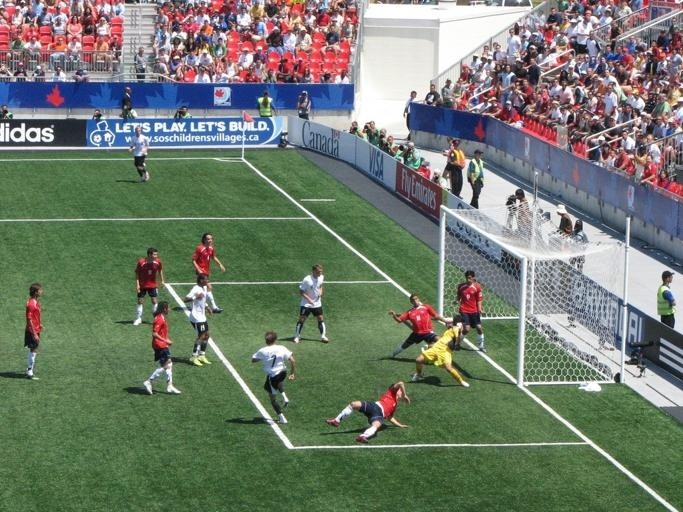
[252,332,295,424]
[23,282,44,380]
[0,105,13,119]
[350,113,591,275]
[256,89,278,117]
[326,381,410,444]
[298,89,311,121]
[129,125,151,183]
[174,106,192,118]
[122,87,132,109]
[656,271,677,328]
[135,232,225,394]
[442,0,683,194]
[92,109,105,121]
[294,264,329,343]
[403,84,441,140]
[119,103,137,119]
[388,270,483,387]
[0,1,349,84]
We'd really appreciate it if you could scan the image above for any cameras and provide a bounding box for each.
[364,122,371,129]
[380,133,385,138]
[506,195,517,211]
[435,176,438,180]
[399,145,409,152]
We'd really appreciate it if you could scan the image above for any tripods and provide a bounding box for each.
[505,211,518,230]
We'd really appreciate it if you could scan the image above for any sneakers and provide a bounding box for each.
[284,397,289,407]
[326,419,339,426]
[26,368,39,380]
[166,384,181,394]
[213,306,223,313]
[294,336,301,341]
[144,380,153,394]
[461,380,470,387]
[356,435,369,443]
[133,317,142,326]
[479,345,487,353]
[140,174,149,181]
[412,372,426,381]
[277,415,288,424]
[190,355,212,366]
[320,337,328,343]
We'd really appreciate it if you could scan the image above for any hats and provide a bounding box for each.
[474,149,483,154]
[556,203,567,214]
[662,271,674,279]
